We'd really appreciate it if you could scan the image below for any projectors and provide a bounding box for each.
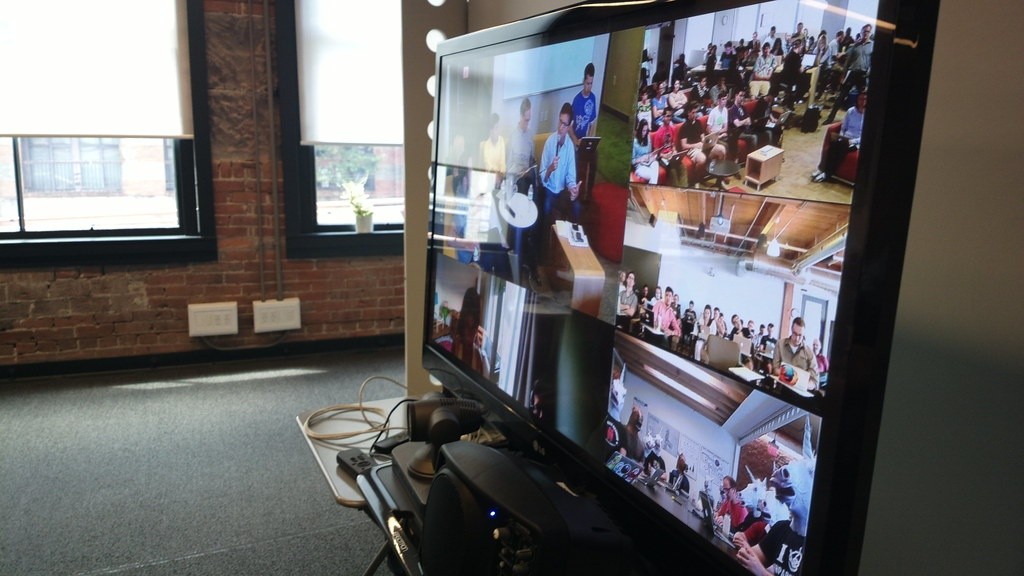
[708,216,732,235]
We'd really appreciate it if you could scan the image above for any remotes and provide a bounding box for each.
[338,447,380,477]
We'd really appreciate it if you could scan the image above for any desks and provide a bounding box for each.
[639,322,665,339]
[296,397,508,576]
[770,375,815,398]
[760,353,773,359]
[706,159,741,191]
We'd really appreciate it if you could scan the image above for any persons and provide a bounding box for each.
[604,413,806,576]
[615,270,830,392]
[452,285,483,376]
[630,20,876,190]
[479,63,598,295]
[528,389,546,422]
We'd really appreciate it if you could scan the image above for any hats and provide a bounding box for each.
[629,410,643,431]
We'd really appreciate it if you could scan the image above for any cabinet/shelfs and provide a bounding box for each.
[744,145,784,191]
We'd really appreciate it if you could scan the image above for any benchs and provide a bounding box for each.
[633,97,758,188]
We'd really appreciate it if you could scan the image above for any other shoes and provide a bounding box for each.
[813,173,826,182]
[812,168,822,177]
[735,174,741,179]
[823,119,832,124]
[723,176,730,184]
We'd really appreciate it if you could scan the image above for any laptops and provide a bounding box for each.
[765,111,792,130]
[661,150,689,168]
[641,146,664,166]
[639,307,664,331]
[744,117,771,133]
[832,132,856,149]
[780,363,811,393]
[733,333,753,357]
[638,468,664,486]
[696,131,721,153]
[573,137,600,159]
[801,53,817,70]
[661,472,684,491]
[700,489,737,549]
[607,452,644,484]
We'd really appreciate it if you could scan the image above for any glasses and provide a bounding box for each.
[722,487,732,491]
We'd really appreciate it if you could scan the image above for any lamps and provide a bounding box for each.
[709,195,731,235]
[766,217,780,257]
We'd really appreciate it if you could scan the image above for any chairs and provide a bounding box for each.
[822,121,859,187]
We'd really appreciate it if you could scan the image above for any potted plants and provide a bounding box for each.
[350,195,374,233]
